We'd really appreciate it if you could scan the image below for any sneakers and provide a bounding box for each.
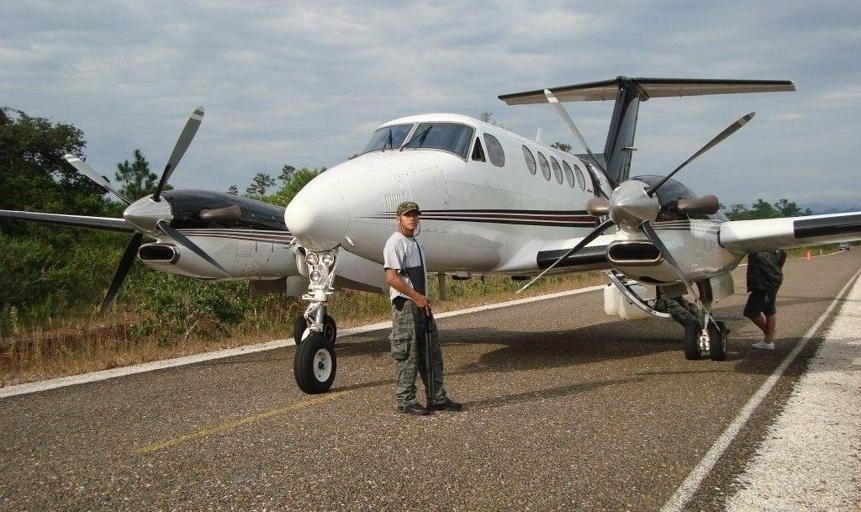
[751,340,776,351]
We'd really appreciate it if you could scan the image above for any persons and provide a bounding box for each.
[743,245,786,350]
[383,201,462,414]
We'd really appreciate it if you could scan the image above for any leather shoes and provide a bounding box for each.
[397,403,430,415]
[427,401,463,412]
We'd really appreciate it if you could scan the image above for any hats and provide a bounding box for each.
[396,200,422,217]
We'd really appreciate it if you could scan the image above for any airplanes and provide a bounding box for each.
[0,76,861,394]
